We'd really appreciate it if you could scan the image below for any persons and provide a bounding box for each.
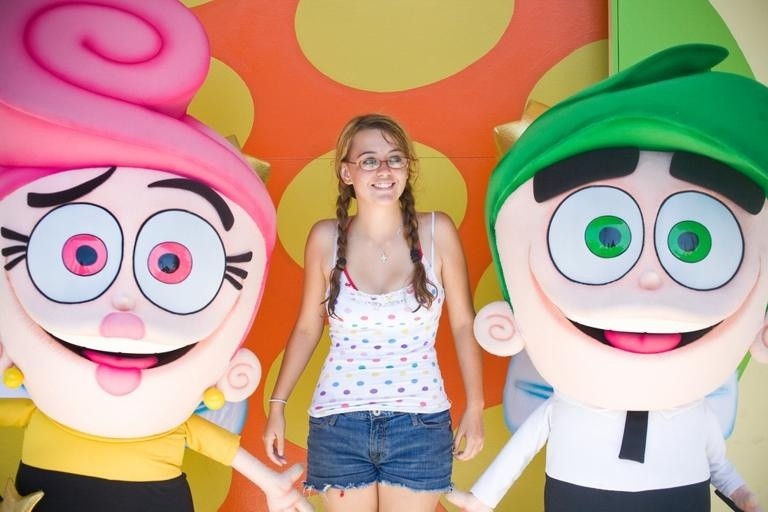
[261,112,487,512]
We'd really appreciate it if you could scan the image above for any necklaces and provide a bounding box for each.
[351,216,403,265]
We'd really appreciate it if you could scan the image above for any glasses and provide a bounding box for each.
[343,156,410,171]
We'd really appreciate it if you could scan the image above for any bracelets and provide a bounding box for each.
[267,398,288,405]
